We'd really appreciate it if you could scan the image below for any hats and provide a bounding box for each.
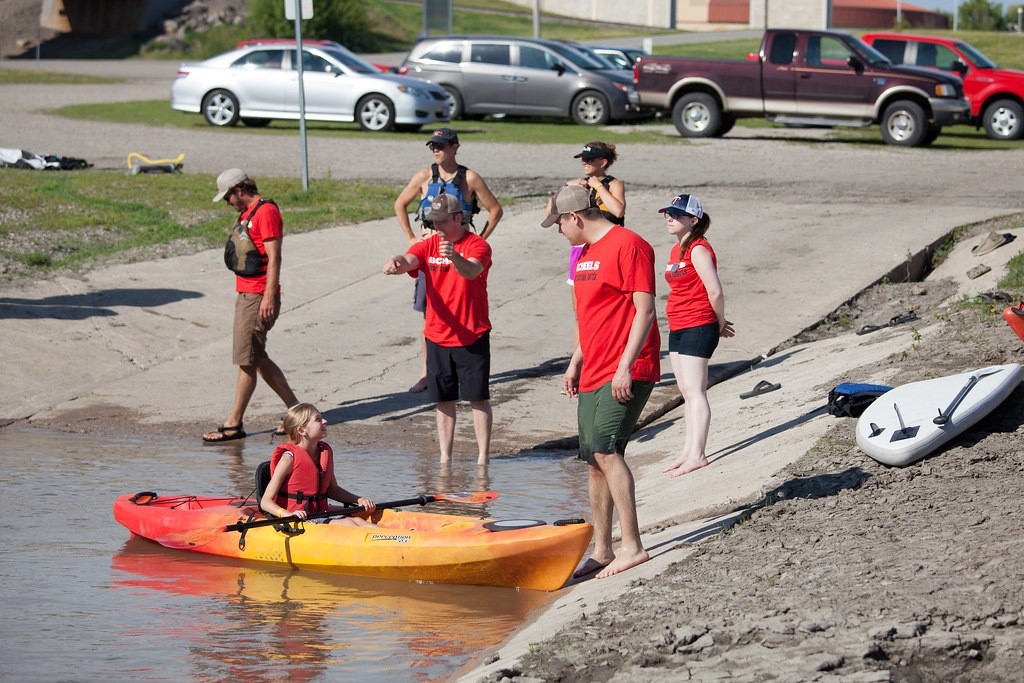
[573,146,609,158]
[541,185,596,228]
[426,128,458,146]
[213,168,248,202]
[659,194,704,219]
[425,193,463,222]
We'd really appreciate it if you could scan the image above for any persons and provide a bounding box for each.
[659,193,736,478]
[540,182,660,579]
[394,128,503,392]
[384,193,493,463]
[546,141,626,395]
[261,402,379,527]
[203,168,299,442]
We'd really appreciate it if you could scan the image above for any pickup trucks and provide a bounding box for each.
[628,29,1024,146]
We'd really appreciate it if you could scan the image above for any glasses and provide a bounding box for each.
[224,185,241,203]
[664,210,696,219]
[428,141,457,150]
[582,157,605,163]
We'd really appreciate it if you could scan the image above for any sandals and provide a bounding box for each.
[203,422,246,442]
[275,424,287,435]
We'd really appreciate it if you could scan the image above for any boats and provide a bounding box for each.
[1003,301,1024,343]
[115,492,594,593]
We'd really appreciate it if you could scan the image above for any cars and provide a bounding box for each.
[171,38,454,131]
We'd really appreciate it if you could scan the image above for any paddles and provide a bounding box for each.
[153,489,499,550]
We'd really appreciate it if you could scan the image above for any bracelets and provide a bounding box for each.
[277,508,285,518]
[595,184,602,189]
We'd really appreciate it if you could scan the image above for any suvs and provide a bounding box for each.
[400,34,663,127]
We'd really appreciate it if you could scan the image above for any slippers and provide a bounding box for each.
[856,325,885,335]
[740,380,781,399]
